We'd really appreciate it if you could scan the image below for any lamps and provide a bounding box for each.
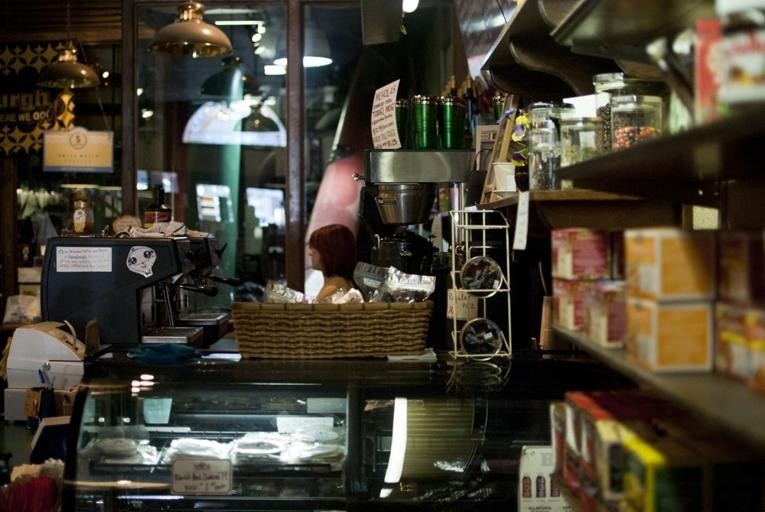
[148,2,333,102]
[28,36,102,92]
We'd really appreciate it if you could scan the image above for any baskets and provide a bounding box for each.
[231,299,432,355]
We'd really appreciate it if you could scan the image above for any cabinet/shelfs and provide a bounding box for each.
[60,356,640,510]
[475,1,764,512]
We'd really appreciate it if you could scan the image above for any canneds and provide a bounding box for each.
[72,199,95,234]
[144,208,171,228]
[528,72,665,191]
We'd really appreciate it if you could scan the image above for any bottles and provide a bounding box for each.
[69,200,95,237]
[527,92,663,188]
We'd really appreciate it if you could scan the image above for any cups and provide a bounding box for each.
[397,95,468,150]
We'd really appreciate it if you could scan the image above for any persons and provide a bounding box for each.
[262,223,285,277]
[308,224,357,304]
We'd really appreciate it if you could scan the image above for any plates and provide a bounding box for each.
[235,429,348,471]
